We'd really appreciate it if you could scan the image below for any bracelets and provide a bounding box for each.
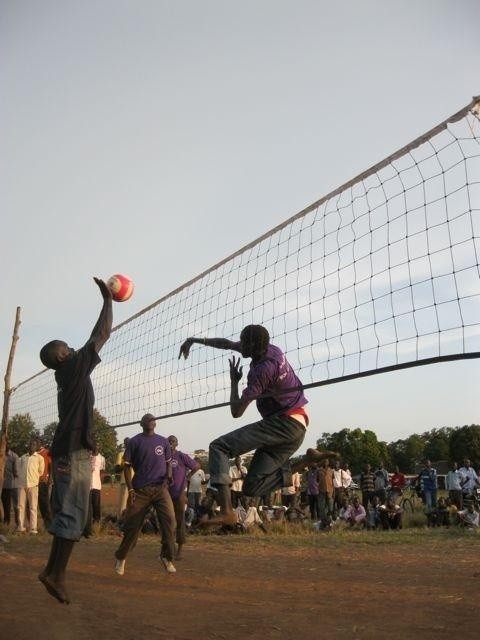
[202,337,208,346]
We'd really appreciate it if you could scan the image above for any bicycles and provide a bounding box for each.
[382,486,414,514]
[333,487,360,512]
[410,485,425,505]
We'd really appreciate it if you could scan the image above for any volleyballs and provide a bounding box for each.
[107,274,135,302]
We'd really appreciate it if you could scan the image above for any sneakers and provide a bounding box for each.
[30,529,38,534]
[38,571,65,603]
[18,525,23,532]
[158,555,176,574]
[42,576,71,604]
[114,558,125,577]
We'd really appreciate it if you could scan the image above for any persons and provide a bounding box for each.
[0,437,221,535]
[177,322,309,529]
[162,434,201,562]
[113,413,178,578]
[36,274,113,608]
[230,456,480,533]
